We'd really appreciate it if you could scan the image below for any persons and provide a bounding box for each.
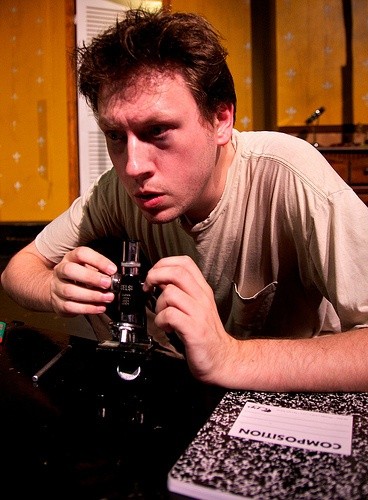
[0,10,368,392]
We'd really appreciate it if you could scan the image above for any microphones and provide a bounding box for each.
[306,107,324,123]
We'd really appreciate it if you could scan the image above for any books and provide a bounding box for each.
[168,390,368,500]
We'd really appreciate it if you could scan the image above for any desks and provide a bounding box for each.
[0,325,227,500]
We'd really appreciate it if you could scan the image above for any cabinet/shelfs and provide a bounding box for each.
[317,145,368,208]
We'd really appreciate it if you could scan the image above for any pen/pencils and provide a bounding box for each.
[32,345,72,382]
